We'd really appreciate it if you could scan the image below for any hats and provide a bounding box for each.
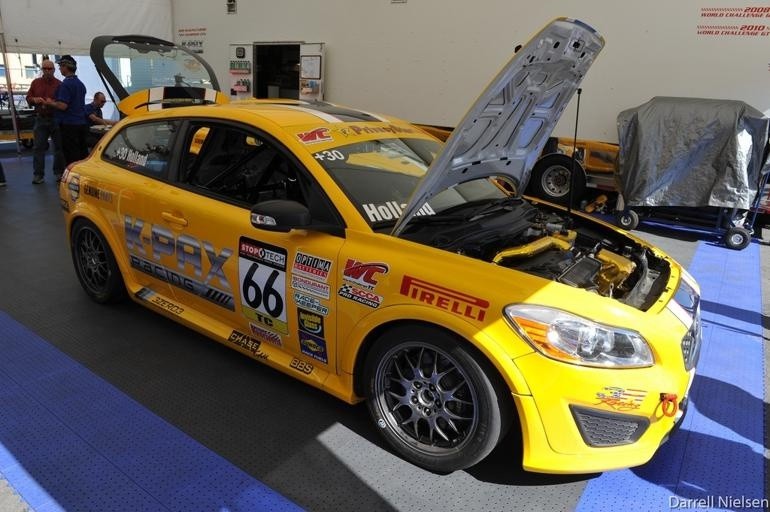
[58,15,703,482]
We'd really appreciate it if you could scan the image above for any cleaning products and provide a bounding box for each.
[614,115,770,251]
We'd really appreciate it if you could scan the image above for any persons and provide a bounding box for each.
[84,92,117,126]
[26,60,66,184]
[45,55,89,165]
